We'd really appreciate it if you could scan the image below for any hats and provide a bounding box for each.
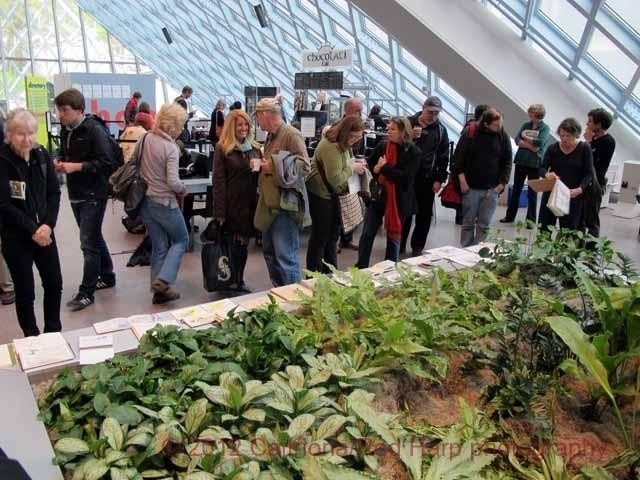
[252,97,281,116]
[425,96,443,113]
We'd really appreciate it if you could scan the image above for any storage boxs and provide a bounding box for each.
[507,183,530,207]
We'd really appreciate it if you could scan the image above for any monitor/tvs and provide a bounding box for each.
[296,110,328,133]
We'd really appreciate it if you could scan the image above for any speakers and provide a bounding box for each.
[161,27,172,44]
[254,4,268,27]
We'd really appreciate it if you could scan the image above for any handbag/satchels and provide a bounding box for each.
[203,243,241,293]
[439,181,461,208]
[109,159,140,201]
[339,192,364,235]
[572,143,605,202]
[124,176,147,220]
[215,125,224,137]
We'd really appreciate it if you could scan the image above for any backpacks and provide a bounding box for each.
[88,119,125,175]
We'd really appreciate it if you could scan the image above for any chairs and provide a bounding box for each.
[188,185,213,249]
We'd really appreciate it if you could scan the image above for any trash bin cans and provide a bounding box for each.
[507,184,528,207]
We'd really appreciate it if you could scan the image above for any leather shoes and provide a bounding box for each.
[412,248,421,257]
[1,290,16,305]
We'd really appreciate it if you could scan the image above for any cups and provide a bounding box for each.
[250,157,261,174]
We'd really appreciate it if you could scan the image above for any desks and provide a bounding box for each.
[182,178,216,251]
[189,138,212,152]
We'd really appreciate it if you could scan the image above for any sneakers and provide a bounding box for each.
[150,279,176,299]
[94,278,115,290]
[151,292,181,305]
[340,241,360,251]
[525,224,535,230]
[500,218,514,222]
[65,295,94,311]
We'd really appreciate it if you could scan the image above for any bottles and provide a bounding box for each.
[191,125,207,140]
